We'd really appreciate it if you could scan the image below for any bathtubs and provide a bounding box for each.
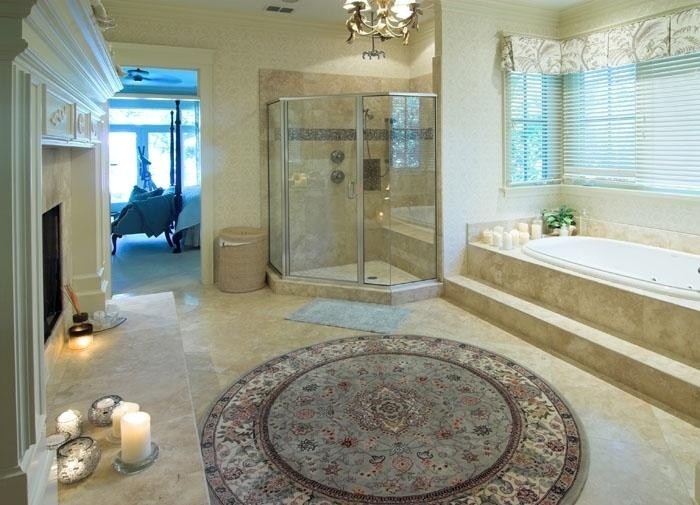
[392,205,436,230]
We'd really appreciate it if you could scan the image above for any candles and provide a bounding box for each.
[97,398,114,424]
[112,401,140,439]
[120,411,150,463]
[58,411,78,437]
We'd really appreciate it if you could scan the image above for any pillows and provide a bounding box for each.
[129,185,148,202]
[134,187,163,200]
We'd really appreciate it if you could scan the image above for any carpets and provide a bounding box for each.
[202,333,590,504]
[284,297,410,334]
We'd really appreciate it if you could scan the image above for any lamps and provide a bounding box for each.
[342,0,423,61]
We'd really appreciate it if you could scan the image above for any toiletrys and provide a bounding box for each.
[580,209,588,236]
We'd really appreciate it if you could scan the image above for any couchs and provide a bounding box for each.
[111,192,178,256]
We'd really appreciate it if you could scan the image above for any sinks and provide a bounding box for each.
[525,234,700,302]
